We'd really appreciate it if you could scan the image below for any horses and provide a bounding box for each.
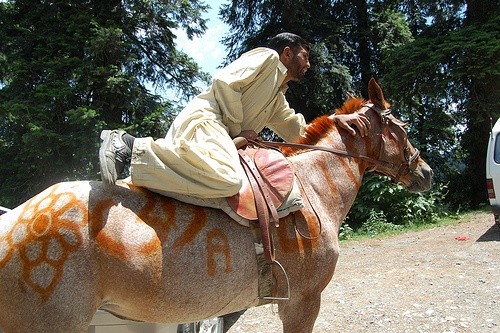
[0,78,433,333]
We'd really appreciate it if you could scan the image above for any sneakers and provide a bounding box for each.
[99,128,133,187]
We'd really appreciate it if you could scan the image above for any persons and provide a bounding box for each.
[99,32,372,199]
